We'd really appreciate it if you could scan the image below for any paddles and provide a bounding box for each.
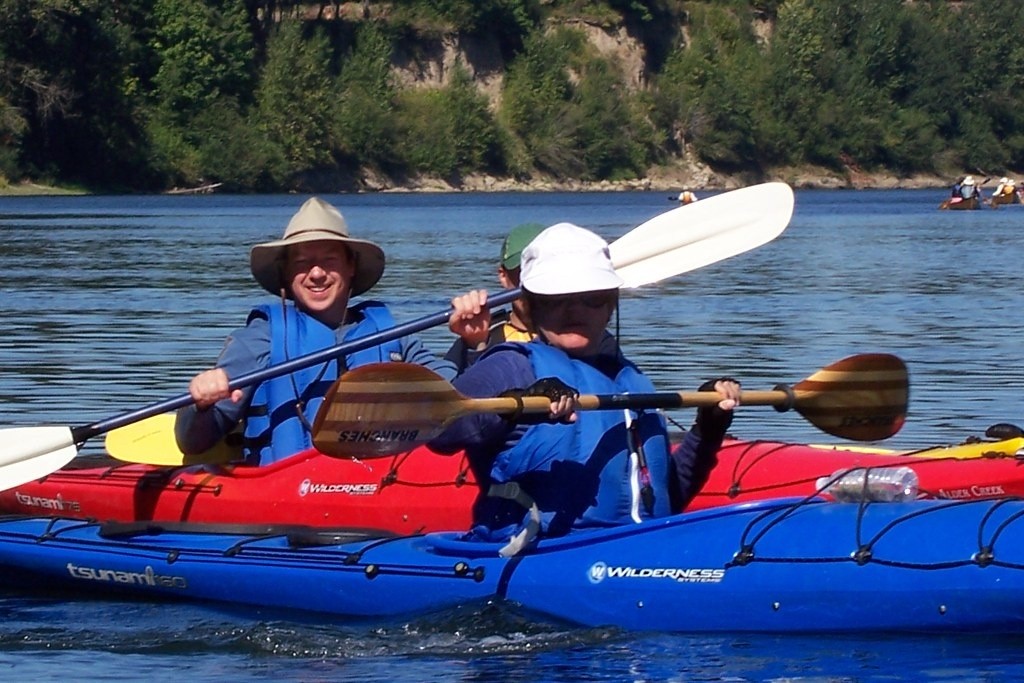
[979,176,991,187]
[311,350,913,463]
[938,200,951,211]
[0,178,799,490]
[667,195,678,201]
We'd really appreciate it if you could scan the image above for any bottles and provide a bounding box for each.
[815,467,919,503]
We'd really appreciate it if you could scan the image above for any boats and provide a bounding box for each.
[0,423,1024,536]
[946,193,980,210]
[0,495,1024,635]
[990,188,1017,205]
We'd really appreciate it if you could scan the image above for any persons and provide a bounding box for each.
[445,221,622,377]
[425,222,741,547]
[174,197,492,467]
[679,186,698,206]
[951,176,1024,201]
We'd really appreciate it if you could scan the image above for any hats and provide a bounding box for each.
[963,176,974,186]
[520,223,624,293]
[1000,177,1008,183]
[501,222,550,271]
[683,185,688,190]
[250,197,386,301]
[957,175,965,183]
[1007,179,1015,186]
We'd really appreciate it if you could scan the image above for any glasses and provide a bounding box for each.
[538,290,611,308]
